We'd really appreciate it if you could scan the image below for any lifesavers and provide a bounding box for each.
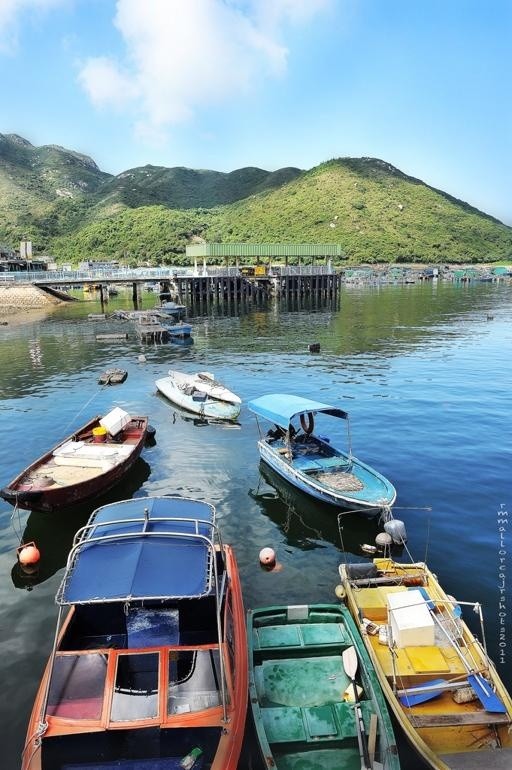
[300,412,313,434]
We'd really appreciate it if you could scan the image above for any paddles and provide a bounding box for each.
[408,586,507,713]
[343,645,380,763]
[401,666,488,707]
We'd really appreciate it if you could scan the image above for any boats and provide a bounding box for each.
[241,604,402,768]
[20,496,249,768]
[158,291,193,345]
[247,394,395,512]
[156,371,242,419]
[1,407,149,511]
[337,559,511,770]
[99,369,126,383]
